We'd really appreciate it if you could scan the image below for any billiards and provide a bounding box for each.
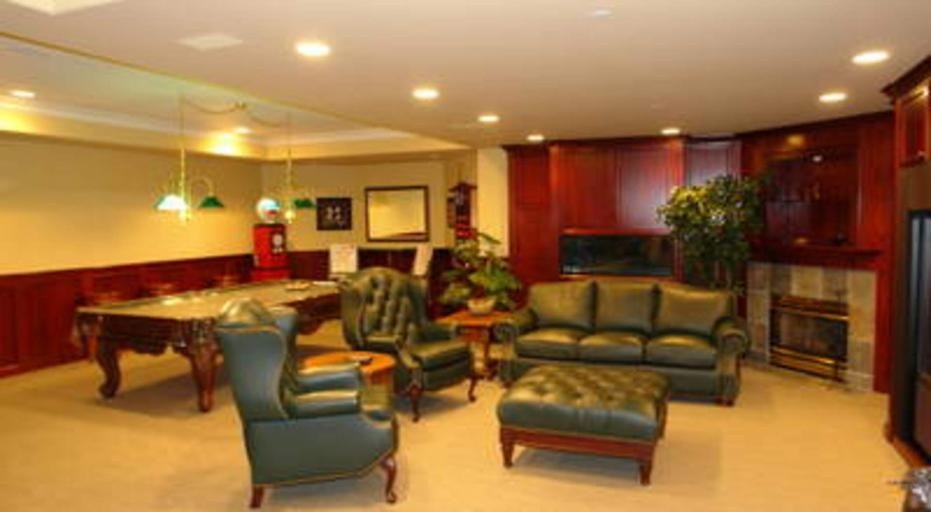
[286,281,308,292]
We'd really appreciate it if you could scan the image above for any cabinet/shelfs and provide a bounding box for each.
[499,142,559,282]
[768,292,849,381]
[676,133,743,280]
[544,134,687,234]
[880,56,930,166]
[744,119,893,308]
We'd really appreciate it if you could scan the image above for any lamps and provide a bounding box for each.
[254,120,318,226]
[157,100,223,218]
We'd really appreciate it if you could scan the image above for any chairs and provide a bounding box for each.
[336,267,476,423]
[217,294,399,509]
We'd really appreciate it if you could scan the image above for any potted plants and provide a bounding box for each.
[443,233,520,313]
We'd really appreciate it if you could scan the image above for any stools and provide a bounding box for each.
[493,366,669,486]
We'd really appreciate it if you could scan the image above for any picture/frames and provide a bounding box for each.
[365,186,429,242]
[316,198,351,230]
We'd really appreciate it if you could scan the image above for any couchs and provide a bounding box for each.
[497,281,749,405]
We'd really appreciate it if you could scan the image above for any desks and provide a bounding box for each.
[77,275,341,413]
[439,305,512,380]
